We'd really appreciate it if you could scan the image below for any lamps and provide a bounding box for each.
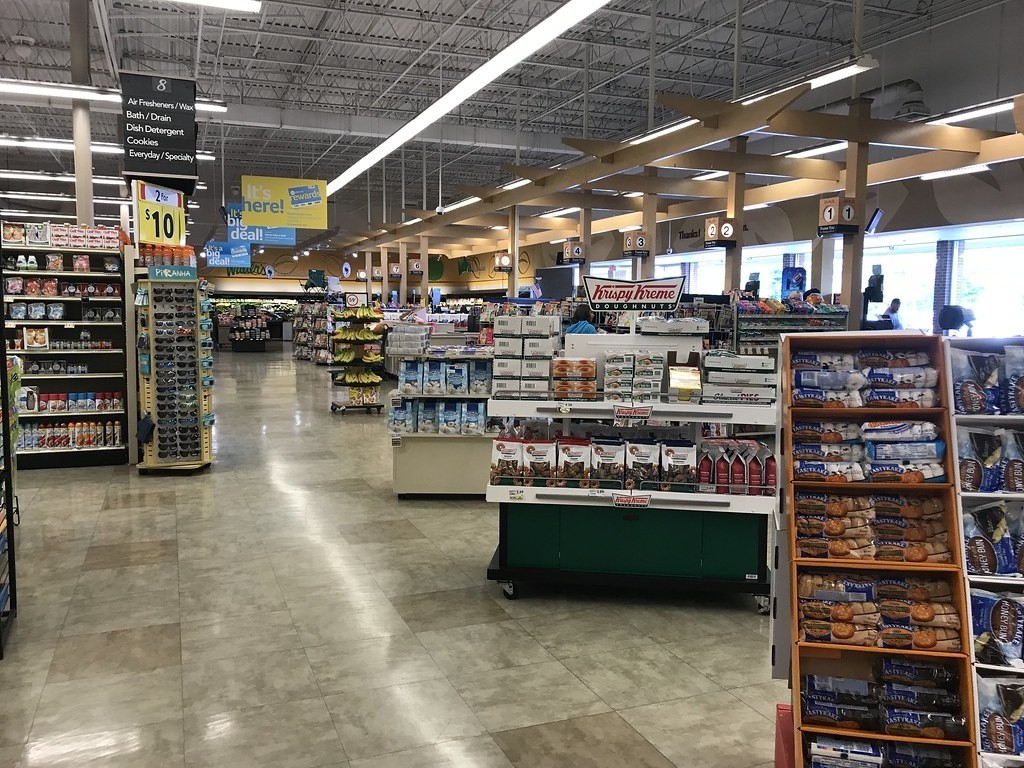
[303,247,312,257]
[0,0,881,221]
[199,250,206,259]
[292,252,301,260]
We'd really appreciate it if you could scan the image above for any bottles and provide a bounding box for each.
[39,392,124,412]
[16,421,122,449]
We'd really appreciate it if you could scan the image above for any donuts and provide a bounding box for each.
[552,360,596,399]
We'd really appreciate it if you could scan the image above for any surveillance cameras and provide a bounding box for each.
[666,248,673,255]
[436,207,443,216]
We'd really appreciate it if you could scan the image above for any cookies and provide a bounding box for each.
[824,357,945,739]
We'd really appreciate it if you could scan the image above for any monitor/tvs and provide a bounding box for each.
[745,281,760,301]
[869,275,884,303]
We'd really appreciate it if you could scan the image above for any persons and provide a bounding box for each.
[885,298,901,329]
[479,304,501,323]
[564,304,597,333]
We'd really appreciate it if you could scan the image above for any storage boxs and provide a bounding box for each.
[492,315,563,400]
[666,350,703,404]
[23,327,49,350]
[19,386,38,413]
[702,354,778,403]
[552,357,598,402]
[25,361,66,374]
[603,349,664,403]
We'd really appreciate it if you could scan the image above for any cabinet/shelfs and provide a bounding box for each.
[772,330,1024,768]
[210,297,850,497]
[0,222,138,471]
[483,397,776,616]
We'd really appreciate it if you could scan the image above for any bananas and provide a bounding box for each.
[328,303,385,384]
